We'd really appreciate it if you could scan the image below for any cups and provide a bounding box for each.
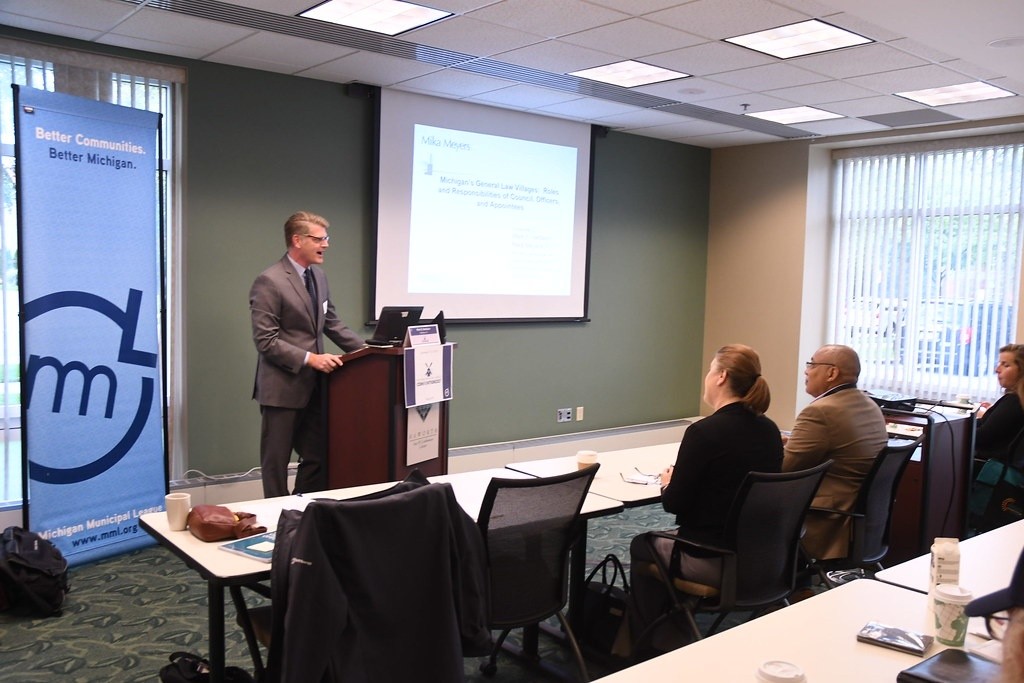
[931,583,975,648]
[165,493,190,531]
[955,394,971,414]
[577,450,597,480]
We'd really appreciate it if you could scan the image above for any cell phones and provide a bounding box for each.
[857,621,935,658]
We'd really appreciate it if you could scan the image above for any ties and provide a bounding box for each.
[304,268,317,325]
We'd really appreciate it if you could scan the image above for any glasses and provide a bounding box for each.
[805,361,835,370]
[298,233,329,243]
[984,610,1024,642]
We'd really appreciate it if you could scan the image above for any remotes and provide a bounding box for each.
[365,338,390,346]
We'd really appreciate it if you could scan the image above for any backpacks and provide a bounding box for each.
[0,526,72,617]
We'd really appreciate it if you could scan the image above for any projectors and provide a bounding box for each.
[865,388,918,416]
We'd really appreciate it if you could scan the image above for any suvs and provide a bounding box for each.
[900,297,1015,375]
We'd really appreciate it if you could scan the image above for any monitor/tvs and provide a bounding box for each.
[371,306,424,342]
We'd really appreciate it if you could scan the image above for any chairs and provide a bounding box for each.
[204,433,927,683]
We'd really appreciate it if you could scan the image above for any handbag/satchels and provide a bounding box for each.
[561,554,632,659]
[187,504,267,542]
[159,651,252,683]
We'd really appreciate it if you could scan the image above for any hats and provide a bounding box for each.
[962,546,1024,617]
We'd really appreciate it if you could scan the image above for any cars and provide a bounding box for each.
[842,294,907,364]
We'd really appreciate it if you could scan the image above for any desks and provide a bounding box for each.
[504,443,682,508]
[874,516,1024,620]
[584,578,992,683]
[138,467,625,683]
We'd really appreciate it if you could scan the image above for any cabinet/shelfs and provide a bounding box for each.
[881,398,980,573]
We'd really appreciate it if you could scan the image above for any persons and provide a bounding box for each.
[964,548,1024,683]
[973,343,1024,503]
[249,212,364,498]
[781,344,888,603]
[631,344,785,662]
